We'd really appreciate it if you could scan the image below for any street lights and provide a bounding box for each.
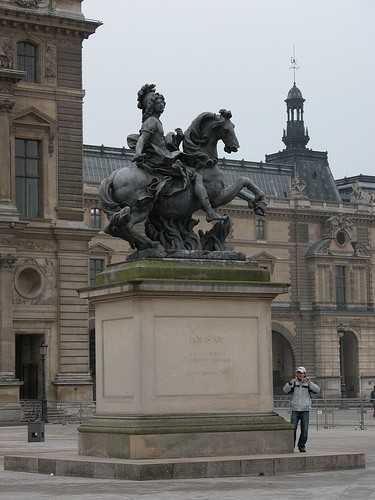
[38,340,48,424]
[337,322,348,410]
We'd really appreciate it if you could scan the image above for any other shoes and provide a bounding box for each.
[298,447,306,453]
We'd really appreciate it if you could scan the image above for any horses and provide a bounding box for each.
[97,109,267,250]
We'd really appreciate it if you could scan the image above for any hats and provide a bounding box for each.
[297,366,306,374]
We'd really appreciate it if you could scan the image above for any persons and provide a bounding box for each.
[282,366,321,452]
[131,91,228,223]
[370,385,375,417]
